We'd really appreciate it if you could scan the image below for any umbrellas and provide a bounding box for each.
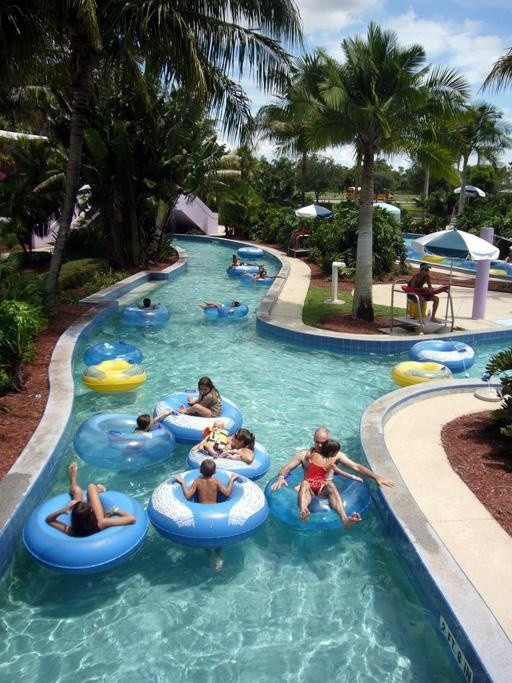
[410,226,501,329]
[454,183,489,205]
[294,200,335,223]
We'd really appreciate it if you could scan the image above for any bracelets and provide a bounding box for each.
[352,473,356,481]
[278,475,286,480]
[110,504,119,516]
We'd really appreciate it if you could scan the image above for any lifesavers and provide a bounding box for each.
[152,389,242,446]
[408,340,475,373]
[390,361,453,387]
[240,274,275,286]
[83,339,143,366]
[228,264,259,277]
[121,304,170,326]
[81,358,147,393]
[187,440,270,480]
[204,300,249,320]
[264,469,372,531]
[147,469,268,547]
[73,412,175,470]
[237,246,265,258]
[21,489,151,573]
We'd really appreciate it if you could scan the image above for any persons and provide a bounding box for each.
[269,424,398,531]
[197,299,240,319]
[127,376,259,464]
[230,255,289,282]
[135,297,159,310]
[43,459,136,539]
[173,458,240,574]
[404,262,443,326]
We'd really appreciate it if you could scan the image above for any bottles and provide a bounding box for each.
[427,309,431,321]
